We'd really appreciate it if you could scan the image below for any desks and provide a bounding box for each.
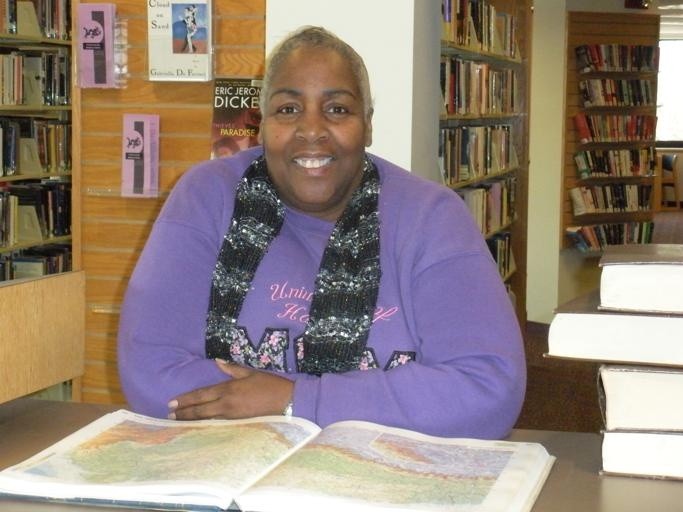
[0,398,682,512]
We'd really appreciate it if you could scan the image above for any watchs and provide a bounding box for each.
[282,399,293,415]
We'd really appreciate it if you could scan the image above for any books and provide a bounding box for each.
[597,242,683,316]
[438,124,520,186]
[0,406,556,511]
[0,42,70,106]
[0,111,72,175]
[485,232,516,278]
[568,185,651,215]
[577,79,653,108]
[660,154,676,207]
[439,53,519,119]
[441,0,522,63]
[0,176,71,246]
[505,281,517,309]
[456,174,517,234]
[564,221,655,252]
[574,145,654,179]
[574,43,655,73]
[0,242,72,281]
[598,428,683,483]
[596,364,683,433]
[209,78,264,159]
[572,110,658,144]
[145,0,211,82]
[0,0,71,39]
[542,289,683,368]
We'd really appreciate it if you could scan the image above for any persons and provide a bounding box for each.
[116,25,527,441]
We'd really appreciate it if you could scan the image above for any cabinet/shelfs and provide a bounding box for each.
[0,0,83,408]
[439,0,529,311]
[561,14,663,260]
